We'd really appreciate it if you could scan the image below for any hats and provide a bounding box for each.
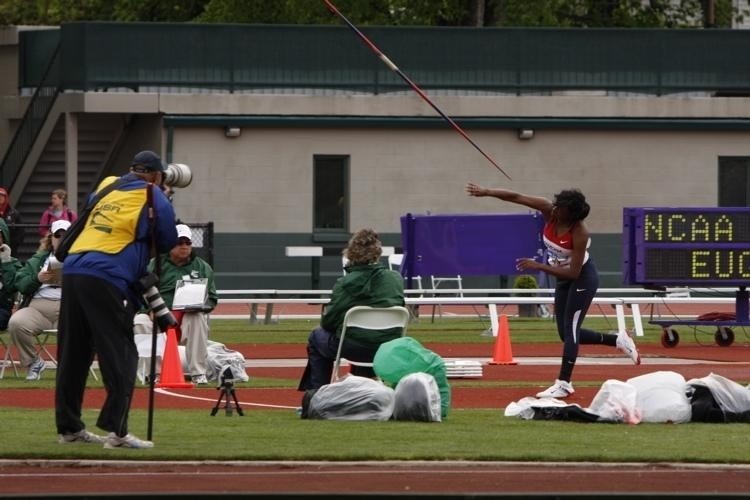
[51,220,72,234]
[175,224,192,240]
[128,150,168,173]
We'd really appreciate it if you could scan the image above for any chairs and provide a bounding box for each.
[35,330,98,382]
[0,291,20,379]
[330,305,409,384]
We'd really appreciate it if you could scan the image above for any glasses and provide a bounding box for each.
[177,241,192,245]
[54,234,61,238]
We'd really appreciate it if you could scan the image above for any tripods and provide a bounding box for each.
[210,378,244,417]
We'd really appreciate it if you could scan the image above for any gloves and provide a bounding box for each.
[0,244,12,263]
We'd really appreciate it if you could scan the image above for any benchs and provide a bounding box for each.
[216,289,737,337]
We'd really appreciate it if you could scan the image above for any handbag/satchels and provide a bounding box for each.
[54,209,90,262]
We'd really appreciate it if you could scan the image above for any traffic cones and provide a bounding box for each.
[488,314,517,366]
[153,327,194,388]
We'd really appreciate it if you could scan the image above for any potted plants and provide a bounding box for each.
[514,275,539,316]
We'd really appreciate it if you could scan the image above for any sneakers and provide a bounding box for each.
[148,375,160,384]
[535,379,575,398]
[103,432,154,449]
[58,430,104,444]
[616,330,640,366]
[191,374,208,384]
[25,357,46,380]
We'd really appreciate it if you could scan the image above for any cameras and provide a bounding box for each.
[162,163,193,188]
[135,272,178,333]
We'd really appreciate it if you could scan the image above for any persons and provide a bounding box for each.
[465,180,641,398]
[0,186,27,257]
[131,224,219,385]
[7,219,73,382]
[37,188,78,239]
[294,228,405,417]
[0,216,25,335]
[55,150,177,450]
